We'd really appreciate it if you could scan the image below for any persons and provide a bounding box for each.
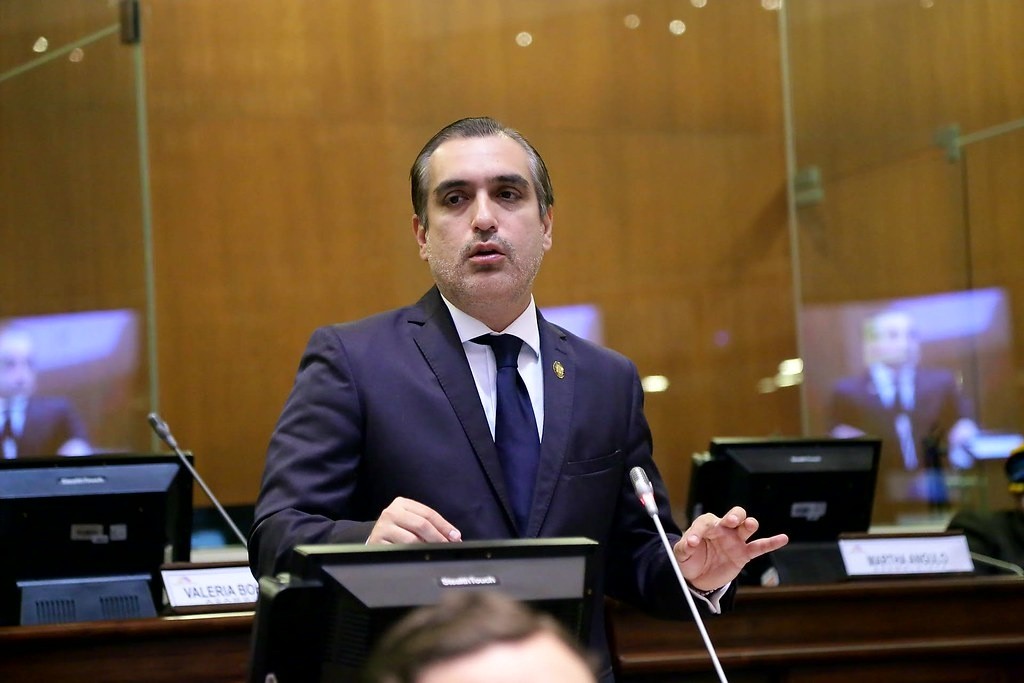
[365,587,608,683]
[0,329,99,456]
[247,114,792,619]
[829,313,977,477]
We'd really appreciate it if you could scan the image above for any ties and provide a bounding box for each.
[468,332,541,537]
[894,375,905,416]
[3,409,13,438]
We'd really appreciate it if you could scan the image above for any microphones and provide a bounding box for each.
[148,412,248,550]
[630,467,728,683]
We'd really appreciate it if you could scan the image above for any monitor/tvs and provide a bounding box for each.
[254,535,599,683]
[699,434,883,588]
[0,450,195,617]
